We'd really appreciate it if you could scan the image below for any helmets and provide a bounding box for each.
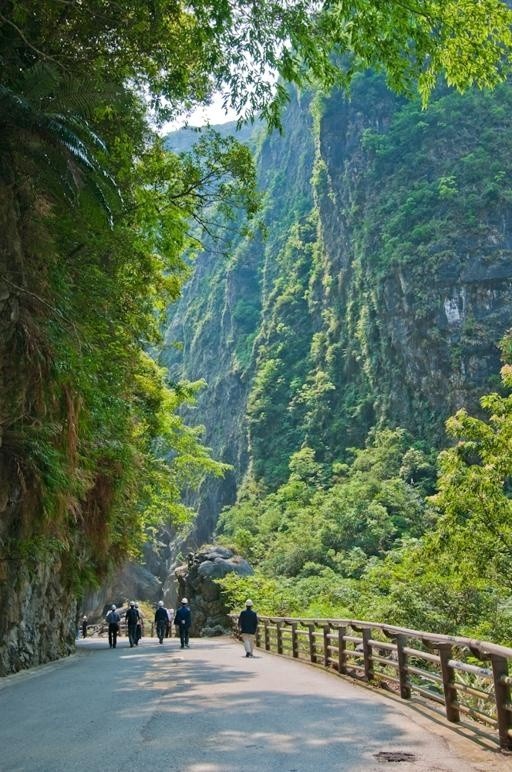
[181,598,188,603]
[246,599,253,606]
[130,601,139,608]
[112,605,116,610]
[159,601,163,606]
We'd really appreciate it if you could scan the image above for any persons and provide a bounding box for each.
[155,601,169,643]
[125,601,139,647]
[174,598,191,648]
[162,608,172,638]
[106,605,120,648]
[82,616,87,638]
[134,603,145,640]
[238,599,258,657]
